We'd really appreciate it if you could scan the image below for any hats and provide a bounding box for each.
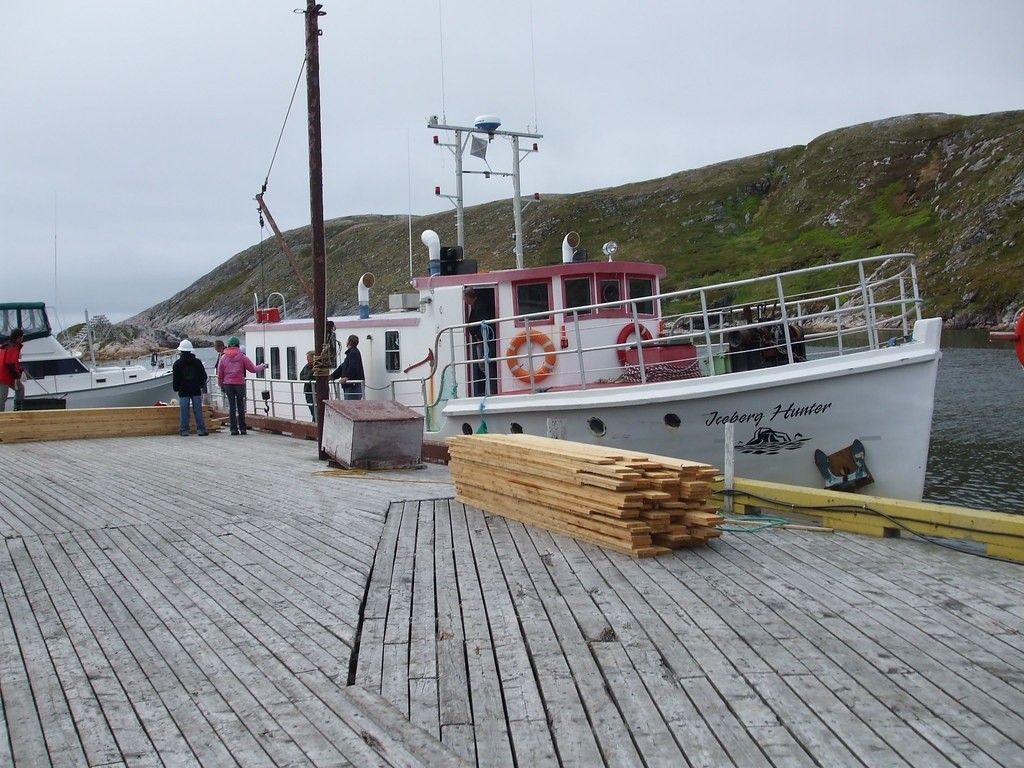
[227,336,240,346]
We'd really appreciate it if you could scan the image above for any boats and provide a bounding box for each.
[0,302,177,412]
[201,114,946,503]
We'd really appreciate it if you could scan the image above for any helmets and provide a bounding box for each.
[177,340,194,351]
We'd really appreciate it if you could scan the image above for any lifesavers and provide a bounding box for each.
[617,323,653,367]
[506,330,556,382]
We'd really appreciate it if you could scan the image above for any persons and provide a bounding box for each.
[172,339,210,437]
[463,286,498,396]
[300,351,316,424]
[328,335,365,400]
[213,335,265,435]
[0,328,27,412]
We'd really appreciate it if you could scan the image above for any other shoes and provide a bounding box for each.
[181,434,188,436]
[198,432,209,436]
[241,432,247,434]
[231,431,239,435]
